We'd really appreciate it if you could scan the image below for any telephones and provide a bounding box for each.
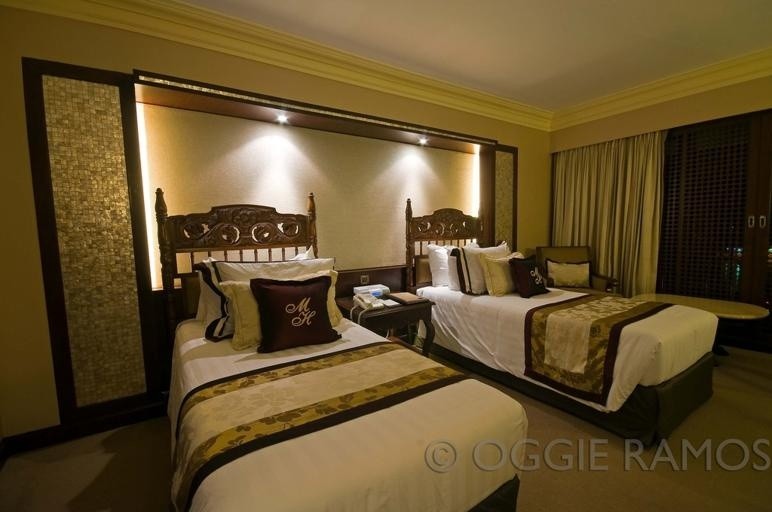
[353,291,384,311]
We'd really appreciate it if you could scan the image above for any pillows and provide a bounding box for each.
[190,244,346,355]
[426,240,591,300]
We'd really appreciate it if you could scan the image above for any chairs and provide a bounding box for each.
[535,246,620,294]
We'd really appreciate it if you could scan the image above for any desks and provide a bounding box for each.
[630,292,770,368]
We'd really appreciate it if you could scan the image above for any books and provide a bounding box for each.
[386,292,425,304]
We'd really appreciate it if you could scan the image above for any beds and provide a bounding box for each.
[154,187,528,512]
[336,295,437,358]
[403,197,719,449]
